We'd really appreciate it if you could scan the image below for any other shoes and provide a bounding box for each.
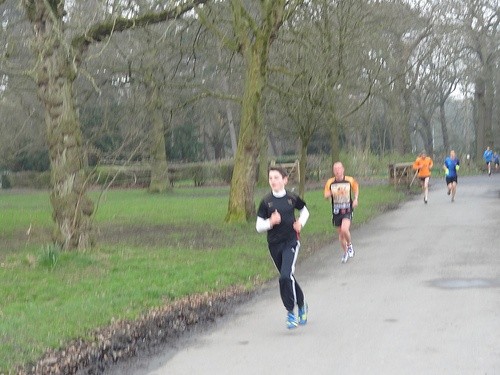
[341,254,348,263]
[286,312,297,329]
[425,200,427,204]
[347,243,354,257]
[451,199,454,201]
[447,191,450,195]
[298,303,308,323]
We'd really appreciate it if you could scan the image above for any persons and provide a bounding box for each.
[256,165,310,328]
[493,150,500,168]
[412,151,433,204]
[482,145,494,176]
[442,149,459,203]
[324,160,359,264]
